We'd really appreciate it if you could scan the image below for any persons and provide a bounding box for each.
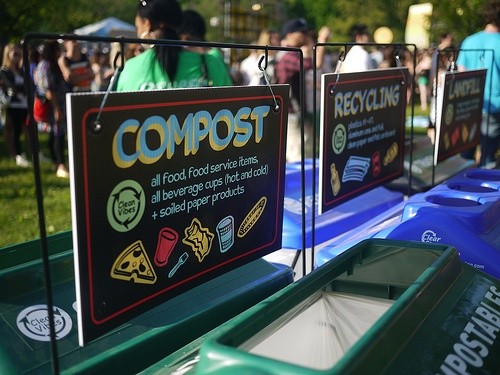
[0,0,455,177]
[456,0,500,169]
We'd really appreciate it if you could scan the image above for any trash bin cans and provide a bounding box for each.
[318,168,500,283]
[0,229,292,375]
[262,132,478,281]
[142,235,500,375]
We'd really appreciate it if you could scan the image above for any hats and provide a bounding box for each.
[280,19,312,35]
[347,24,372,39]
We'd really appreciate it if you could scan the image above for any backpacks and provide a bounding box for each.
[249,50,288,87]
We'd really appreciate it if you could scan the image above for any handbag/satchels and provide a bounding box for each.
[33,97,53,124]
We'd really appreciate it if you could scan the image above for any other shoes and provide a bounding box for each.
[57,168,69,178]
[16,156,31,167]
